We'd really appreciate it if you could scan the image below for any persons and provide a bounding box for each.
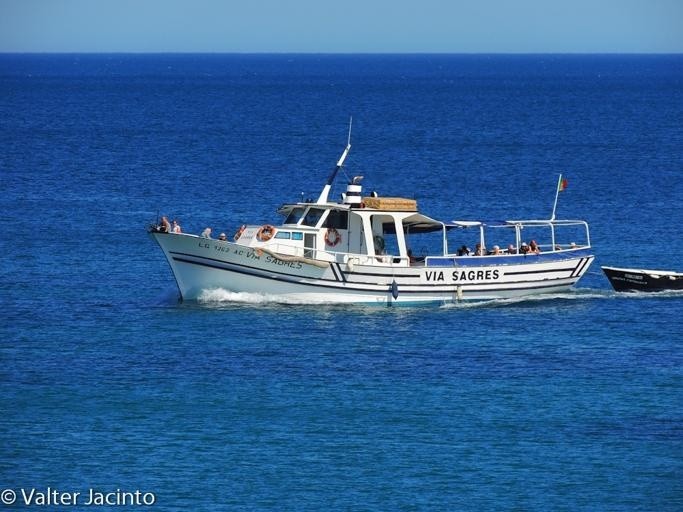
[160,217,171,233]
[571,242,577,249]
[457,240,540,255]
[201,228,211,239]
[220,233,227,241]
[173,220,181,233]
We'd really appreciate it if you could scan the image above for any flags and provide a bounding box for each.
[559,179,568,192]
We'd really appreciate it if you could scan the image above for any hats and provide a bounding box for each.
[521,242,527,246]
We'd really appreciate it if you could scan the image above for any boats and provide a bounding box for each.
[146,114,593,309]
[600,265,683,297]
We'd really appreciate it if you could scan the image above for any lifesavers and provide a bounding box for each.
[234,225,246,240]
[325,229,340,247]
[258,225,275,241]
